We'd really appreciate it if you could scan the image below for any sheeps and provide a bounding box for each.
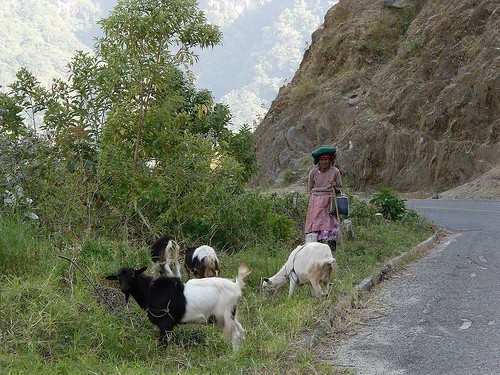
[260,241,338,300]
[151,235,182,282]
[105,260,253,356]
[184,245,221,279]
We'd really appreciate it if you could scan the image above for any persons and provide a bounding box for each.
[305,147,343,253]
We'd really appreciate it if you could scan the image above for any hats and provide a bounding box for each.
[312,146,337,165]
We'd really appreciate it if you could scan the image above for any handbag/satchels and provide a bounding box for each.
[328,191,348,215]
